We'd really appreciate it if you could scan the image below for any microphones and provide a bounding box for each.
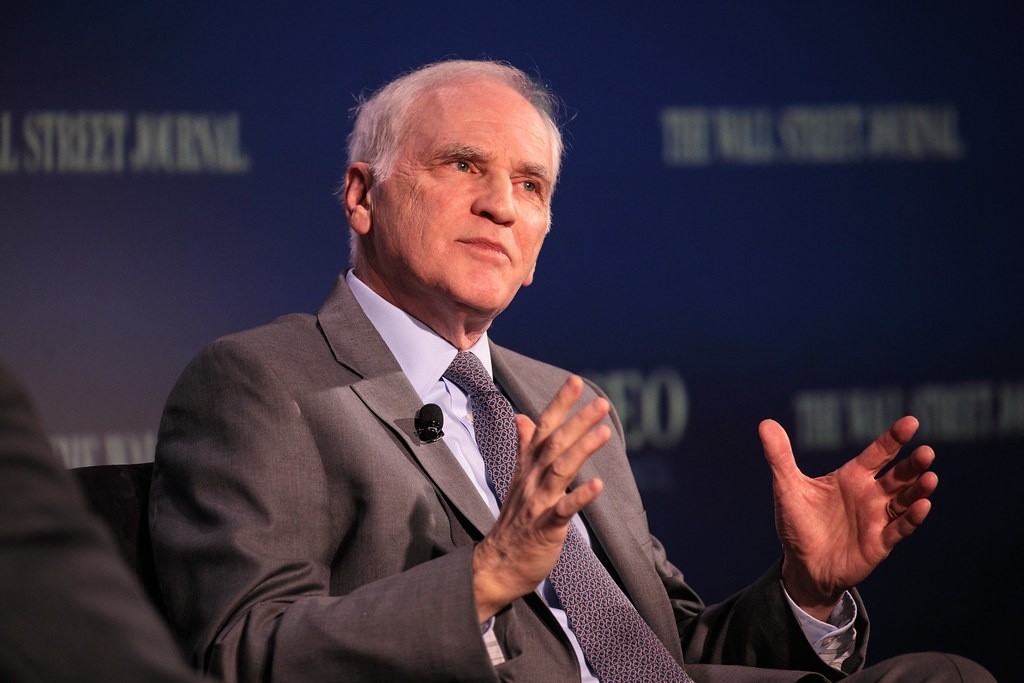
[417,404,444,443]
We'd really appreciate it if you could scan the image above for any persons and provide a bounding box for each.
[0,357,193,683]
[154,61,999,683]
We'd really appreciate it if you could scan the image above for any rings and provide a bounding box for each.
[887,502,898,518]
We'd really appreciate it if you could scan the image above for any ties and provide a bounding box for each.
[442,352,695,683]
